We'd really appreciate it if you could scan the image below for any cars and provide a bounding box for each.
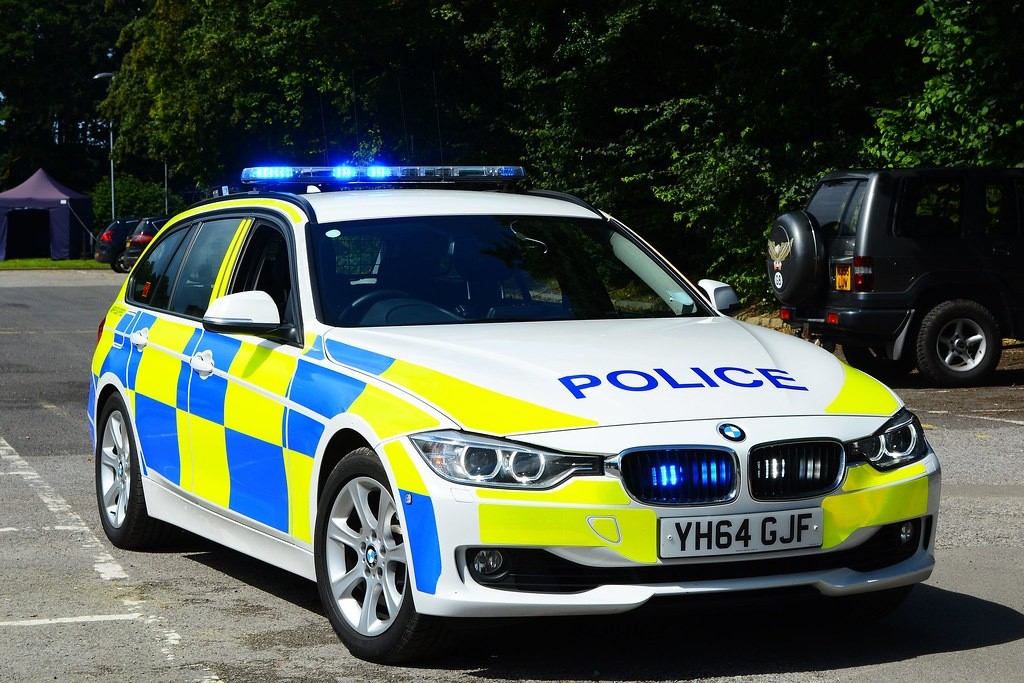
[84,163,945,671]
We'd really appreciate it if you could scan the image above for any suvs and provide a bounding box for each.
[93,216,174,273]
[767,165,1023,388]
